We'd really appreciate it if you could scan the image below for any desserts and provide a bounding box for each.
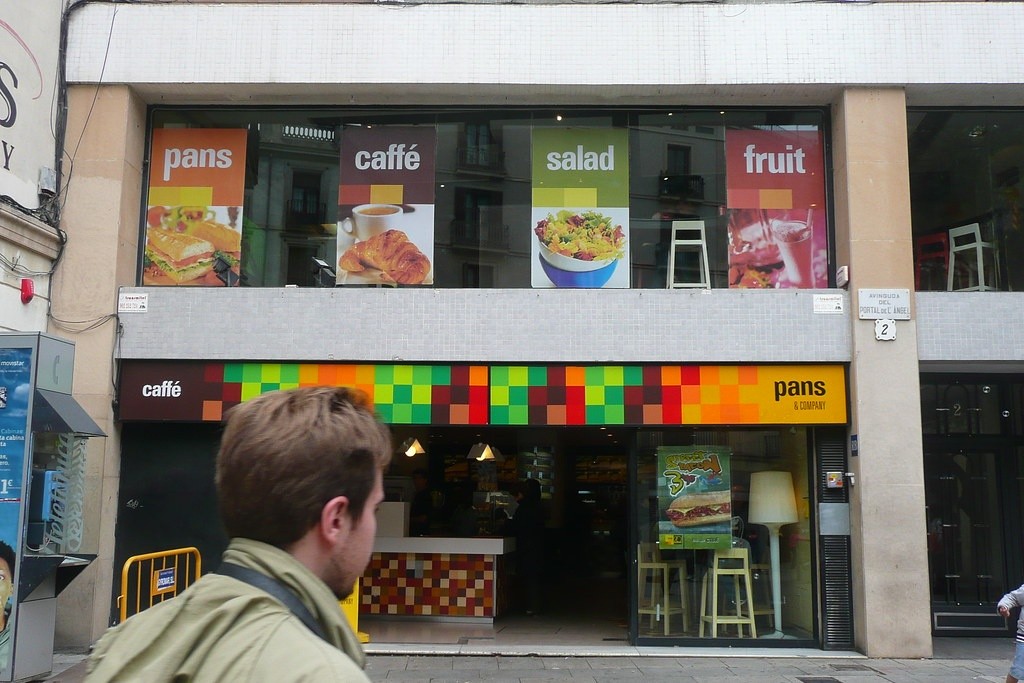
[729,207,786,287]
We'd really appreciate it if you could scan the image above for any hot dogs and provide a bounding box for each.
[667,490,730,526]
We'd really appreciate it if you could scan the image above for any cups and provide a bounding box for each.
[770,213,816,288]
[341,204,403,241]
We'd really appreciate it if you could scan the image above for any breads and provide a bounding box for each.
[339,230,430,283]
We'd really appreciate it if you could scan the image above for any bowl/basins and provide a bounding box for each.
[538,237,618,288]
[321,224,336,234]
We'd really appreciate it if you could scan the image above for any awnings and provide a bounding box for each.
[33,389,109,439]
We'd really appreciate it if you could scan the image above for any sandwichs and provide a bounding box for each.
[146,219,241,284]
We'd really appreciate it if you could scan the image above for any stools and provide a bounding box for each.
[698,548,756,640]
[915,233,968,291]
[636,545,688,636]
[667,220,711,291]
[723,562,774,632]
[946,223,1000,293]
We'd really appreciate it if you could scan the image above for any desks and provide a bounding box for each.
[358,537,514,624]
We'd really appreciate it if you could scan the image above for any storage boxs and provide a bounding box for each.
[375,501,410,537]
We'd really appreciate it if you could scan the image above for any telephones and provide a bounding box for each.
[42,468,62,523]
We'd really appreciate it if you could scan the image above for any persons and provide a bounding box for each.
[996,583,1024,683]
[408,468,437,536]
[0,540,16,680]
[498,479,548,615]
[83,384,392,683]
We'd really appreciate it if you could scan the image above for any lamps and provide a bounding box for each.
[397,439,425,458]
[469,445,494,462]
[749,472,799,640]
[211,259,239,287]
